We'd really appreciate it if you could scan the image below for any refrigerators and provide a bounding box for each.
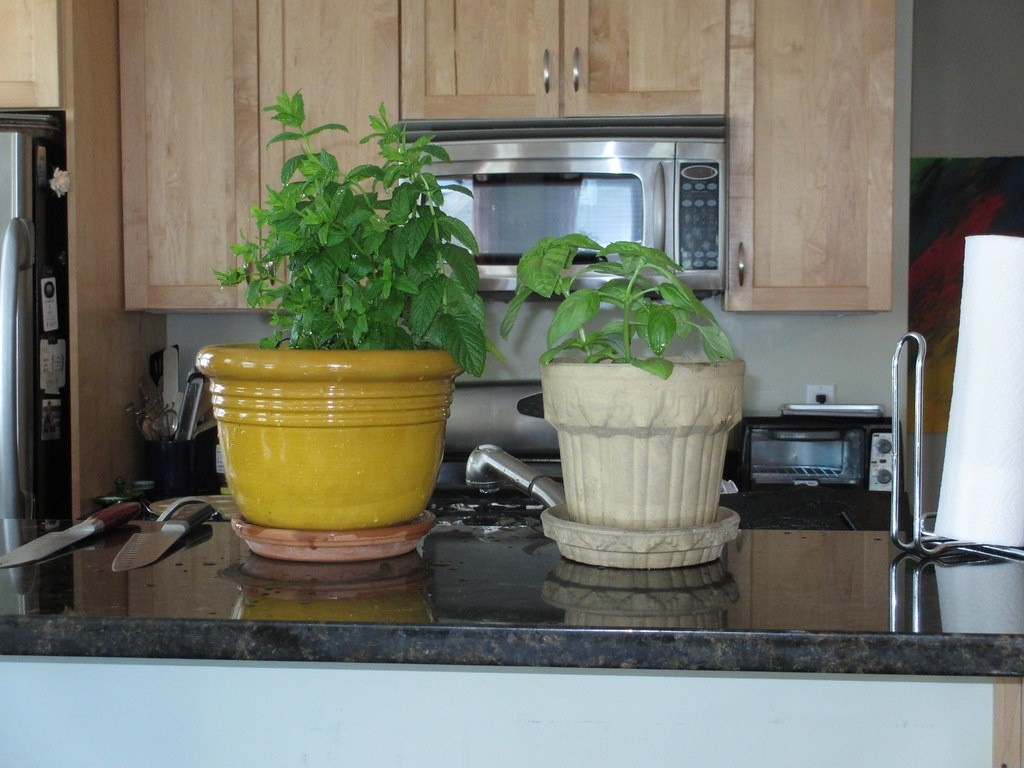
[0,107,70,521]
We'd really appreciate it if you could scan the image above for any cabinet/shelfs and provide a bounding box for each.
[117,0,897,318]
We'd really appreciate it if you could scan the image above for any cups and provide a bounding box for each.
[140,439,196,501]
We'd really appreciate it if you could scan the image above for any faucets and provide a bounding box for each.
[465,443,566,508]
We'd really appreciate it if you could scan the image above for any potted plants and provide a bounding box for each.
[195,88,507,530]
[499,232,746,530]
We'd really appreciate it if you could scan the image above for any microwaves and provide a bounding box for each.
[737,417,904,494]
[393,126,728,298]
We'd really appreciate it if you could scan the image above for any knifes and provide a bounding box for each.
[0,501,141,563]
[111,502,213,572]
